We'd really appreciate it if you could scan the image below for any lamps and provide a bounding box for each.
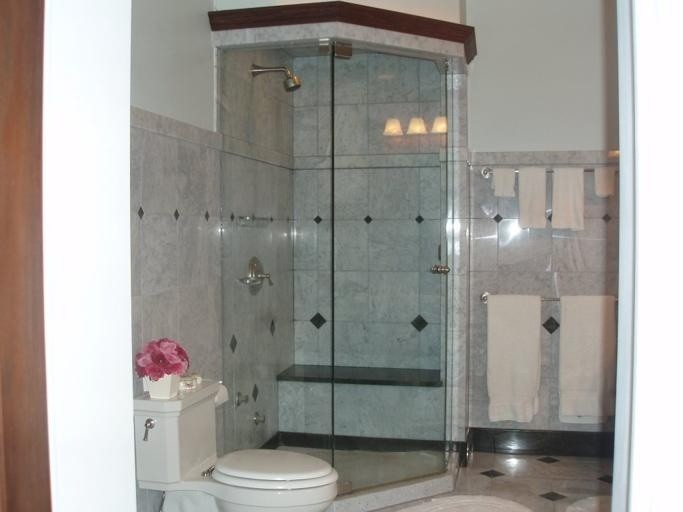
[382,116,448,136]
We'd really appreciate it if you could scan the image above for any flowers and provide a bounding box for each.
[134,336,191,382]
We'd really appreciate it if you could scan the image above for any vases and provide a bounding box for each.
[144,374,181,400]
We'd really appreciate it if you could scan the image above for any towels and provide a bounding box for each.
[485,293,617,425]
[490,165,616,231]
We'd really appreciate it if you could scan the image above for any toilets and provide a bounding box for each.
[133,379,339,512]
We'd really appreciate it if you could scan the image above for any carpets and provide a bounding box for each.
[395,493,612,512]
